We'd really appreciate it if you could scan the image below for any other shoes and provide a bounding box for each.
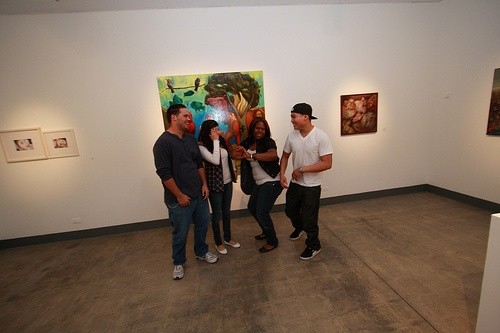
[255,233,267,240]
[173,265,184,279]
[215,244,227,255]
[196,252,218,264]
[290,229,305,240]
[224,240,241,248]
[259,244,278,253]
[300,245,321,260]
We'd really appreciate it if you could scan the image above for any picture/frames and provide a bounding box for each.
[0,128,48,163]
[340,93,378,136]
[41,128,80,158]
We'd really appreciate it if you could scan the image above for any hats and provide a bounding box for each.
[291,103,318,119]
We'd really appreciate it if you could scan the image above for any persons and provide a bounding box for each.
[236,117,284,253]
[280,103,334,259]
[197,119,241,255]
[153,104,219,280]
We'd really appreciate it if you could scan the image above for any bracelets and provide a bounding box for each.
[251,154,252,160]
[298,167,304,174]
[253,153,256,161]
